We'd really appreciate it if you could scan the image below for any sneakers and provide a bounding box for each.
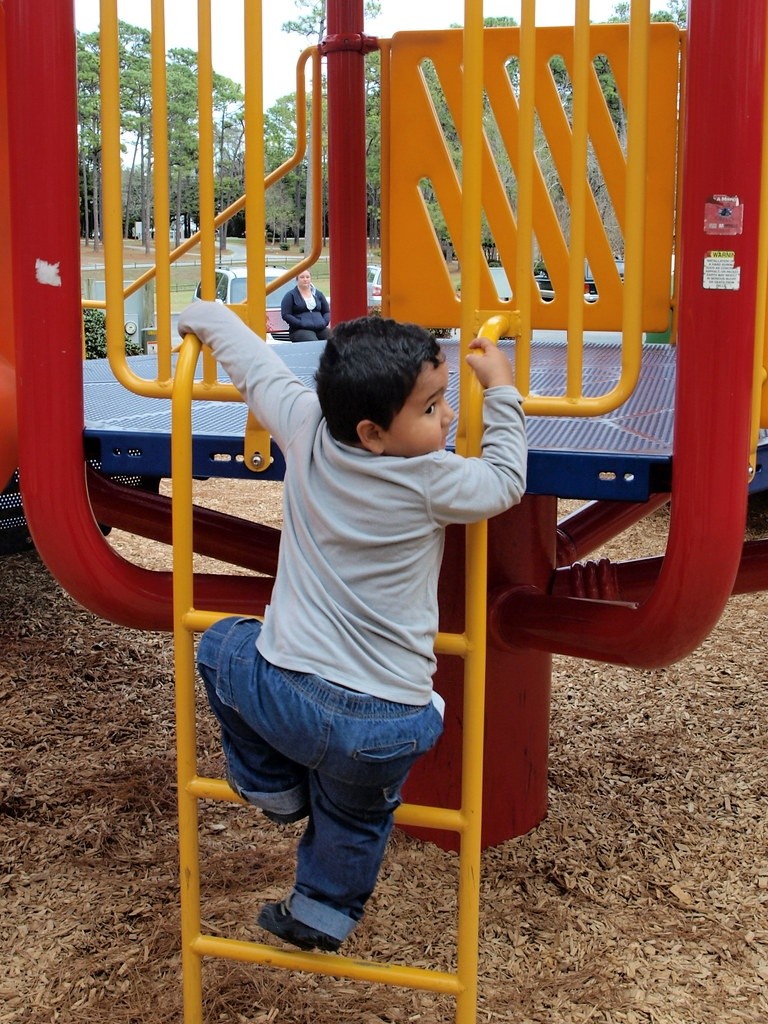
[225,764,309,825]
[258,900,340,952]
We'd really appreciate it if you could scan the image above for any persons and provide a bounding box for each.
[281,272,332,342]
[177,300,530,955]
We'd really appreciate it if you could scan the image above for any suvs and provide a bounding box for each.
[533,255,625,302]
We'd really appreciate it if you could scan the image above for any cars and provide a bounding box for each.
[365,264,383,308]
[489,267,513,303]
[194,266,330,342]
